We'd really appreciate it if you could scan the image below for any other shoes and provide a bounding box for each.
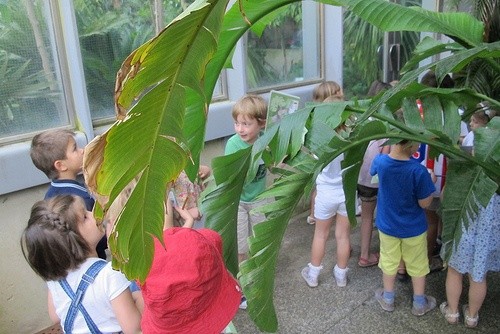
[374,287,395,311]
[332,268,348,288]
[301,267,319,286]
[397,268,407,280]
[410,294,436,316]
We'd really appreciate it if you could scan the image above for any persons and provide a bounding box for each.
[301,80,500,330]
[19,95,277,334]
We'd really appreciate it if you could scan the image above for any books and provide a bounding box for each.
[263,89,301,135]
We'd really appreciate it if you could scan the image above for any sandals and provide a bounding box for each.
[462,304,479,327]
[440,301,459,324]
[359,253,380,267]
[306,216,316,225]
